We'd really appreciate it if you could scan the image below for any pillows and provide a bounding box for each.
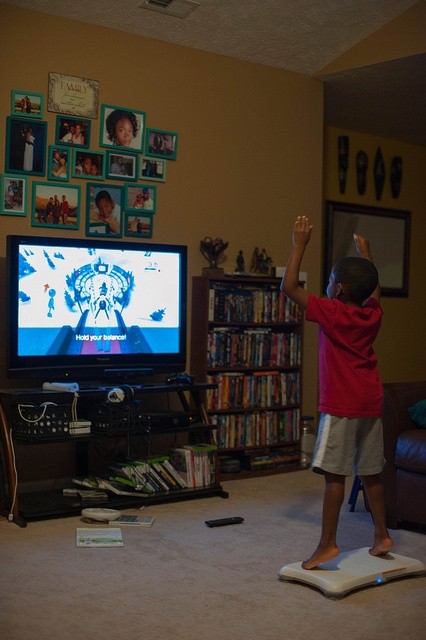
[408,399,425,426]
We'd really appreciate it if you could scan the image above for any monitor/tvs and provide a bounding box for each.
[5,235,187,378]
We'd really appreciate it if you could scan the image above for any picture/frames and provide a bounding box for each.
[99,103,146,154]
[124,182,157,213]
[55,114,91,149]
[47,144,70,182]
[0,173,29,217]
[5,115,48,177]
[105,149,140,182]
[324,200,411,300]
[71,147,105,181]
[11,89,45,119]
[144,127,178,161]
[124,211,154,239]
[31,180,82,230]
[85,182,124,238]
[138,157,167,182]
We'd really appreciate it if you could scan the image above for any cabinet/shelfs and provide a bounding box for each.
[189,274,306,481]
[0,375,231,529]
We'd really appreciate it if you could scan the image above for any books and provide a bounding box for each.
[108,514,156,528]
[62,443,219,501]
[208,284,298,477]
[76,527,124,548]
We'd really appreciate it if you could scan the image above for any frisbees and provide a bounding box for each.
[81,508,121,520]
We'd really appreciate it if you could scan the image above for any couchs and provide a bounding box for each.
[362,381,425,520]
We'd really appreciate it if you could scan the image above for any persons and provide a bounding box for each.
[145,160,157,177]
[106,109,138,147]
[60,195,69,224]
[8,180,23,209]
[90,164,98,176]
[46,197,54,224]
[76,155,91,175]
[23,126,35,171]
[153,133,168,154]
[110,156,128,175]
[52,152,61,172]
[52,195,59,223]
[133,192,144,209]
[137,219,142,233]
[26,98,31,114]
[90,190,121,233]
[280,215,394,570]
[131,216,138,232]
[59,124,75,143]
[21,96,28,113]
[142,188,153,210]
[72,125,84,144]
[52,156,66,178]
[118,163,126,175]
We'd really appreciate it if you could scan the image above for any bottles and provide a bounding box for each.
[298,415,316,470]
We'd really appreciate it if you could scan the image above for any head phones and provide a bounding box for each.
[102,384,134,409]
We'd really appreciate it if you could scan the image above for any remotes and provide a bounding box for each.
[206,516,244,528]
